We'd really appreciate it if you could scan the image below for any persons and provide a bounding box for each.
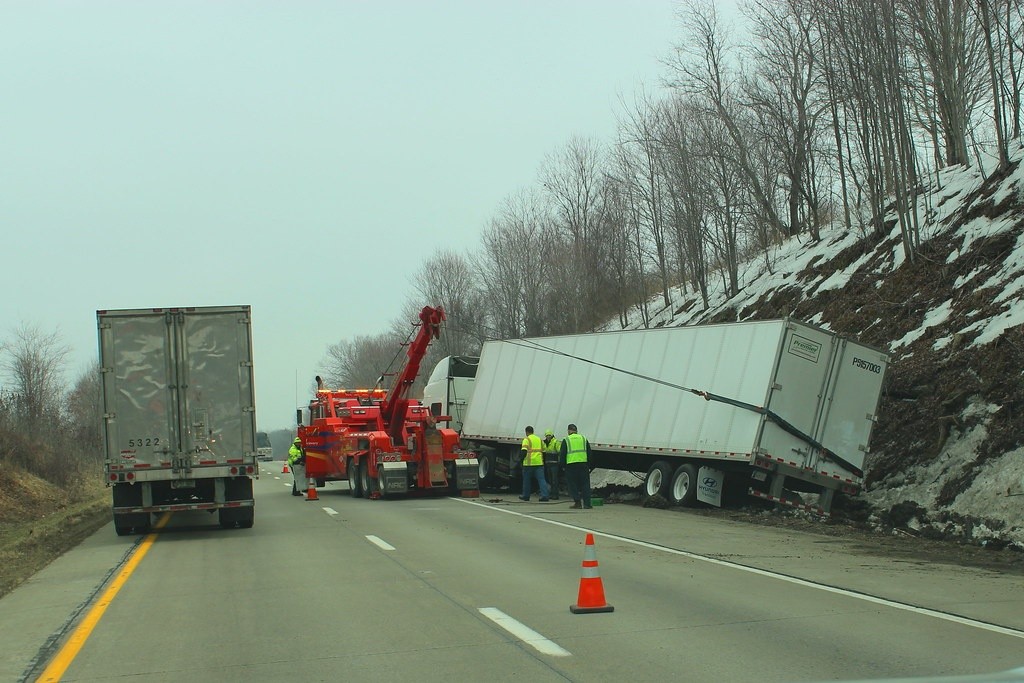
[519,427,548,501]
[289,437,305,496]
[541,430,561,500]
[560,424,595,509]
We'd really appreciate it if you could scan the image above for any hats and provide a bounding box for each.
[294,436,302,443]
[544,429,554,436]
[568,424,577,431]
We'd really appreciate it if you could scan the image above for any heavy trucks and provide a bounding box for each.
[424,317,889,523]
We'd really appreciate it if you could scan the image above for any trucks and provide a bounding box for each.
[256,432,275,462]
[95,304,262,536]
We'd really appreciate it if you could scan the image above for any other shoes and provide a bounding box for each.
[519,495,530,501]
[550,495,559,500]
[292,492,304,496]
[569,502,583,509]
[539,497,550,502]
[583,504,593,509]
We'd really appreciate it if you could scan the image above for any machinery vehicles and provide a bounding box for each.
[295,307,481,499]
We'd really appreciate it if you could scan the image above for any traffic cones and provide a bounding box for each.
[282,460,295,477]
[570,532,620,613]
[305,473,320,501]
